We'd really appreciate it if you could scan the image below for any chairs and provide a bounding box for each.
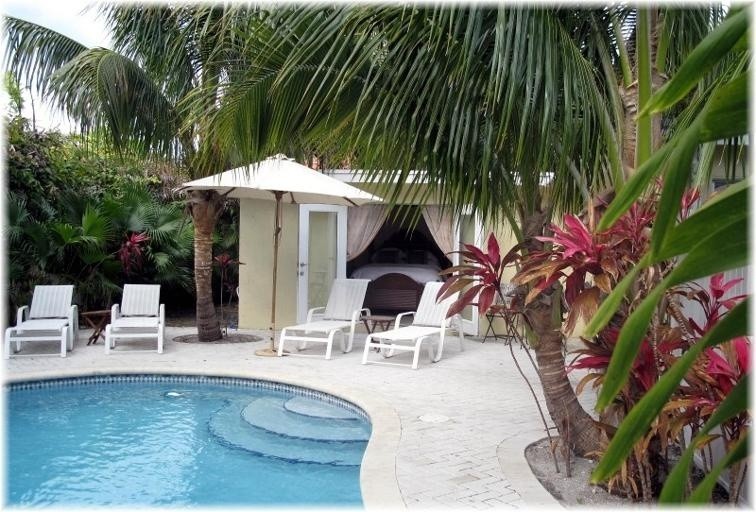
[104,283,165,354]
[275,278,374,360]
[4,284,78,358]
[359,281,466,369]
[483,282,526,345]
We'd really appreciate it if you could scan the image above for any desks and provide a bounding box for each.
[80,310,110,346]
[359,315,395,352]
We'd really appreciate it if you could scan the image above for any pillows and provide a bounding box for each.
[369,246,405,264]
[406,250,439,266]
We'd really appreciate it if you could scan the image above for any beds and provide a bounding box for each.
[348,229,444,313]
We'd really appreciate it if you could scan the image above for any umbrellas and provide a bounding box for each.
[182,152,387,359]
